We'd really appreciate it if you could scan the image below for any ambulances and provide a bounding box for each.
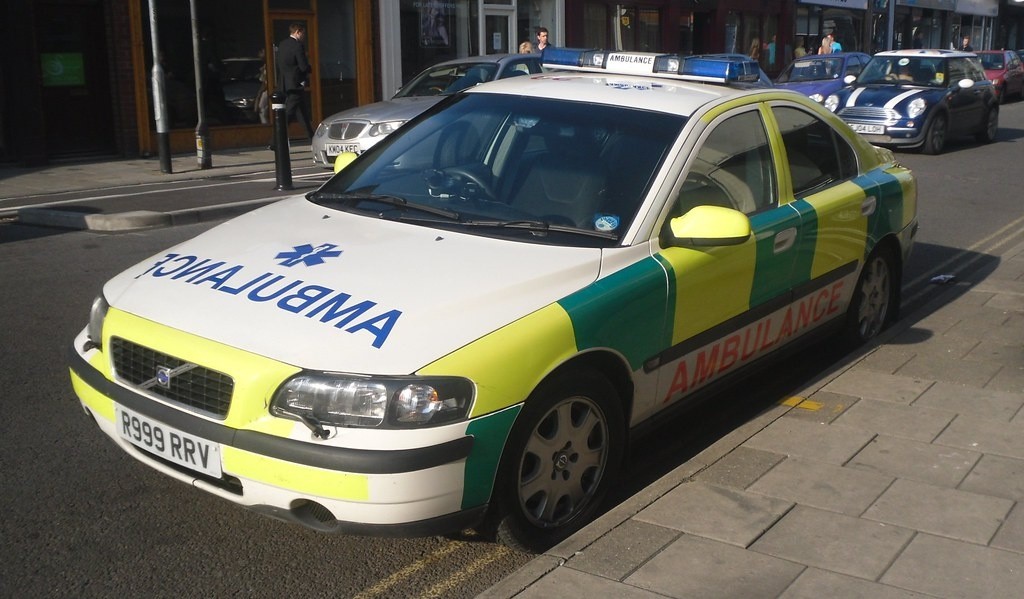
[65,48,922,557]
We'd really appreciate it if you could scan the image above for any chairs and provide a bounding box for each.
[507,117,604,234]
[511,70,528,77]
[463,76,483,89]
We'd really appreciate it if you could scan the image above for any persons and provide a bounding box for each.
[959,35,975,53]
[795,29,842,59]
[198,24,234,126]
[898,63,913,76]
[763,34,775,68]
[913,30,925,49]
[515,41,546,74]
[254,44,269,124]
[535,26,554,55]
[747,37,760,61]
[267,22,313,149]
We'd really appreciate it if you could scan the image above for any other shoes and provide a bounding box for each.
[267,143,274,150]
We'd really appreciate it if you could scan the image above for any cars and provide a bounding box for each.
[688,51,770,90]
[973,49,1023,104]
[772,52,881,121]
[823,48,1002,157]
[310,51,548,170]
[216,54,274,123]
